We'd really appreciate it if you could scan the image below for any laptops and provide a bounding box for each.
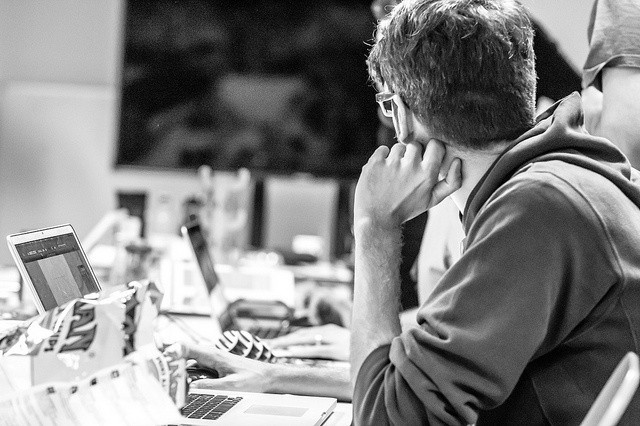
[7,224,337,426]
[182,220,315,340]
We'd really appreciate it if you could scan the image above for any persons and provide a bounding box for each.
[186,1,639,425]
[266,1,583,360]
[582,0,639,171]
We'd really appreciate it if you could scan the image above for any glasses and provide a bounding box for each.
[375,91,410,117]
